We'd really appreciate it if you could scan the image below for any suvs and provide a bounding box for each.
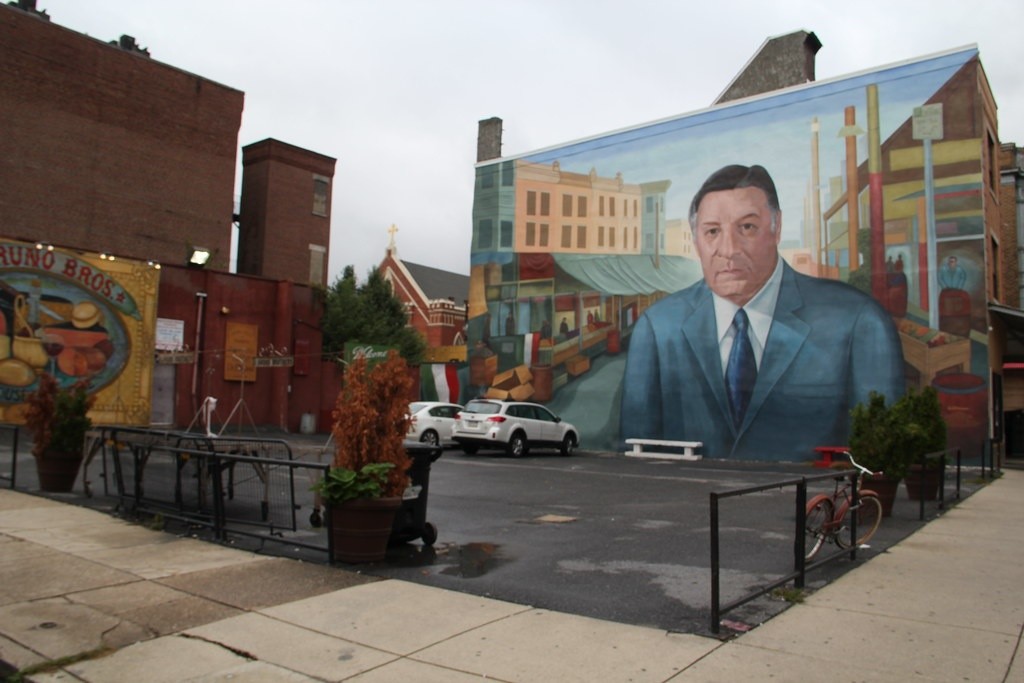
[452,396,581,458]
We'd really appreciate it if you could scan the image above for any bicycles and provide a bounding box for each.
[797,446,885,565]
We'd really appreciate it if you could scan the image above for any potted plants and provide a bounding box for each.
[897,384,955,502]
[322,345,415,569]
[26,374,97,494]
[844,390,913,515]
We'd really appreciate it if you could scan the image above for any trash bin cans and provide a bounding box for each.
[388,439,444,546]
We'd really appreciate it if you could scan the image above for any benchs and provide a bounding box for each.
[813,444,851,468]
[623,437,704,458]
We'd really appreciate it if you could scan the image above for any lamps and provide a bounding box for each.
[189,245,211,266]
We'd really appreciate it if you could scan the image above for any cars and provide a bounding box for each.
[398,402,465,454]
